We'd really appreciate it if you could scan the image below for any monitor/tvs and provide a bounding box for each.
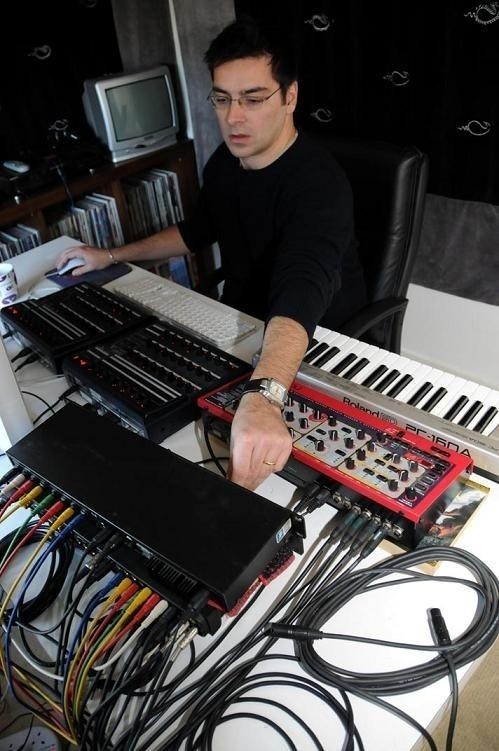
[82,66,180,163]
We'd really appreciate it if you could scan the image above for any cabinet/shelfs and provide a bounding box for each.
[1,147,209,292]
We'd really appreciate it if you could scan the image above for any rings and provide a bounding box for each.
[263,461,276,465]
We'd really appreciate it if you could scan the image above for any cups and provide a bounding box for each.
[0,262,20,306]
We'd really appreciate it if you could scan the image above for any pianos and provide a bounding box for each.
[253,325,499,482]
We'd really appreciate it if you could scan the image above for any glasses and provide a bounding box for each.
[204,84,283,111]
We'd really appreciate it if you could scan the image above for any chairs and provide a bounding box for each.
[332,132,428,354]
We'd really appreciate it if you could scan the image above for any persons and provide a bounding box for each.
[56,16,368,490]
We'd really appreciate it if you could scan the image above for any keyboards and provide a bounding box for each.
[114,278,256,347]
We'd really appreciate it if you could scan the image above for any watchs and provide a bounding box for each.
[239,378,289,413]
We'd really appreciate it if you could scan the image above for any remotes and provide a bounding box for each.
[3,160,30,173]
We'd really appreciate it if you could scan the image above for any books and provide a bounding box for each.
[0,168,201,291]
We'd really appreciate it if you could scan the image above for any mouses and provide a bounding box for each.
[58,257,85,277]
